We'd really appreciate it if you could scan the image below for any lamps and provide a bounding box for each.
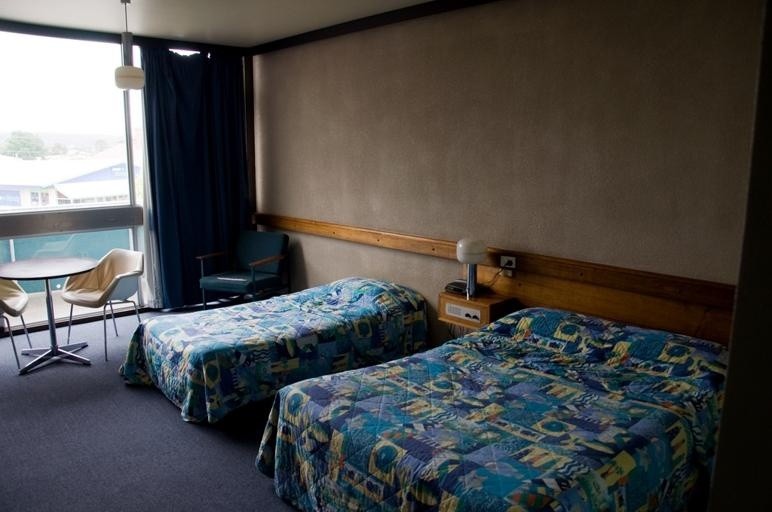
[455,238,490,300]
[114,0,145,89]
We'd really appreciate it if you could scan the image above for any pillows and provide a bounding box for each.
[326,276,416,312]
[478,306,624,364]
[605,324,730,396]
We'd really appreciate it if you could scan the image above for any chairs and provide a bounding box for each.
[0,277,33,370]
[197,229,290,311]
[59,247,145,362]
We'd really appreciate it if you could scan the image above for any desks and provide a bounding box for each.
[0,257,101,376]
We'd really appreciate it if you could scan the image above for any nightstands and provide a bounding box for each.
[438,290,513,338]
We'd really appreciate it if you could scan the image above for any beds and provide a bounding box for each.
[253,304,732,512]
[117,276,431,427]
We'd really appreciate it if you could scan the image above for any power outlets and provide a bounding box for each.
[500,255,516,268]
